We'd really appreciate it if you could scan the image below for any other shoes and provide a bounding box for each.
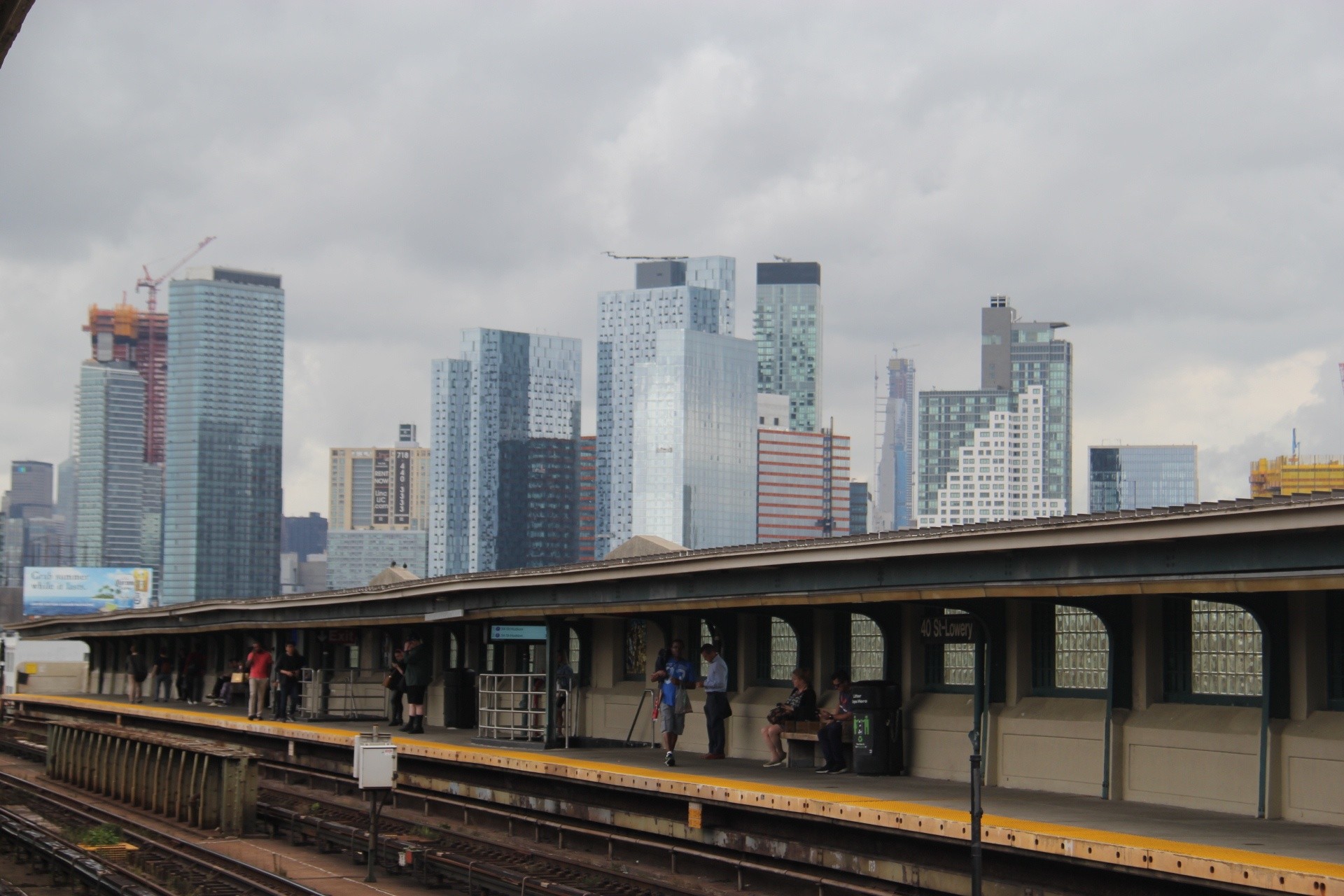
[664,753,674,767]
[700,750,725,760]
[389,718,403,726]
[813,763,848,775]
[208,703,226,708]
[248,714,262,721]
[398,725,424,734]
[206,693,216,699]
[276,716,295,723]
[188,701,198,705]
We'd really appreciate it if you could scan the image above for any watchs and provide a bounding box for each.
[829,714,833,721]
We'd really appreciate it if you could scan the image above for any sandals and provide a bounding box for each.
[763,753,785,766]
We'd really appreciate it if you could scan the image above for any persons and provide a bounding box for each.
[176,640,204,706]
[814,670,852,774]
[125,645,145,704]
[388,631,430,734]
[761,666,816,767]
[695,643,729,760]
[151,649,171,702]
[555,648,573,738]
[651,641,691,767]
[205,639,304,723]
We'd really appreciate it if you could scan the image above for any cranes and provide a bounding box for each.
[136,234,220,464]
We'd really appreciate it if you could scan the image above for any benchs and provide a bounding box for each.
[783,732,853,774]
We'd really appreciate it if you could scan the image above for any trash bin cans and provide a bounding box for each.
[849,679,904,775]
[442,668,476,729]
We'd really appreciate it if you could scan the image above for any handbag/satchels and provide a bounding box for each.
[766,703,787,725]
[673,681,694,715]
[134,668,148,682]
[382,675,394,689]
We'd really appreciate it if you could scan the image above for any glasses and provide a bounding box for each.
[833,682,841,688]
